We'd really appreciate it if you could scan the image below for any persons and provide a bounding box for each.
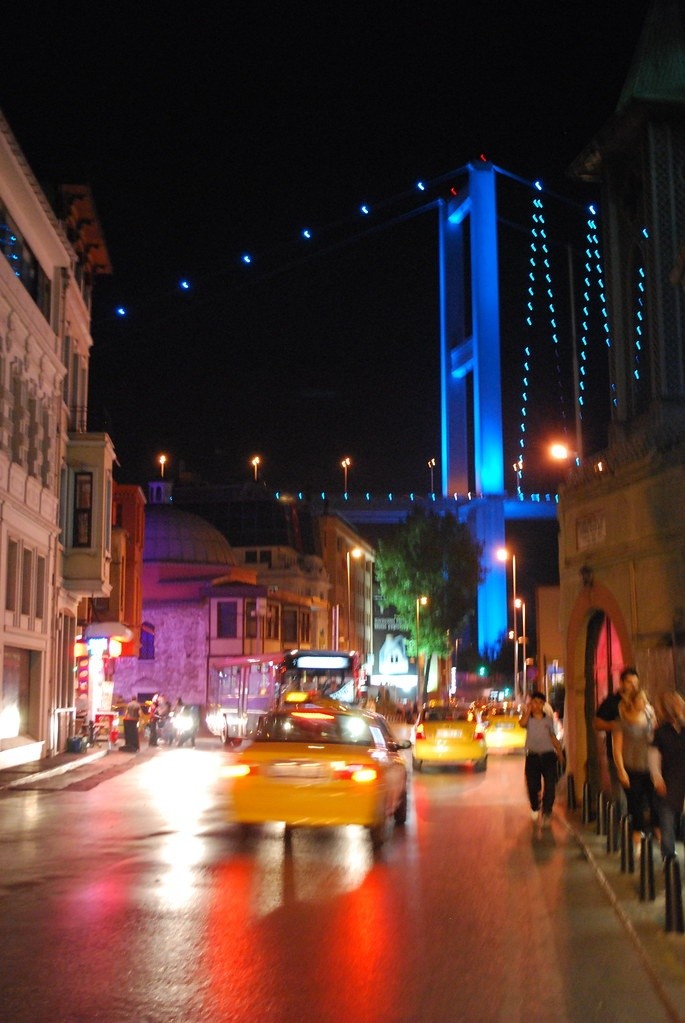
[115,694,199,753]
[518,691,566,828]
[592,667,685,875]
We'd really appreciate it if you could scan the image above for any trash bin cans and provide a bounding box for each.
[123,718,140,752]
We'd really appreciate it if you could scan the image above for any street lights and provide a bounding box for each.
[346,545,365,655]
[513,597,527,701]
[415,595,429,716]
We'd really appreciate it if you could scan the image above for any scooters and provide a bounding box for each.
[160,706,199,747]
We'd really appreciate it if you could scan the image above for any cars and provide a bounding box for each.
[405,703,489,774]
[217,693,412,847]
[477,701,530,755]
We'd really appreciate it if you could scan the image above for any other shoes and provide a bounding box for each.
[632,831,642,859]
[542,812,550,827]
[531,810,539,822]
